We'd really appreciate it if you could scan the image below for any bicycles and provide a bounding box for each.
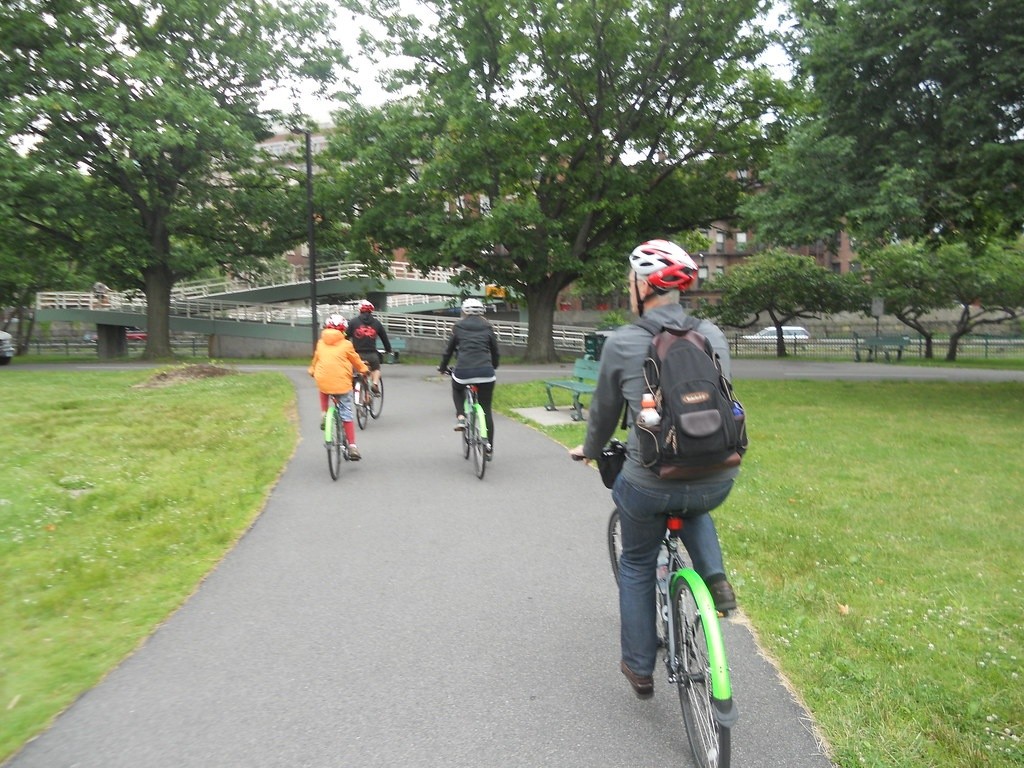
[436,365,492,479]
[570,435,741,768]
[351,349,396,430]
[320,392,360,482]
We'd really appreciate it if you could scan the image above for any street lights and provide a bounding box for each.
[291,125,318,358]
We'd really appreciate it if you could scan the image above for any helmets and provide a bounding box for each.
[359,300,374,313]
[461,297,485,316]
[323,314,348,332]
[628,238,700,292]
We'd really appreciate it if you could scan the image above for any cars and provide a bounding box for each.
[741,326,811,342]
[89,328,149,344]
[0,329,14,366]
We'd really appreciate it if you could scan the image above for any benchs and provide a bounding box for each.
[852,335,912,363]
[376,337,407,364]
[539,357,603,421]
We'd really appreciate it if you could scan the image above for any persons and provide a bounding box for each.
[307,314,370,458]
[345,302,391,393]
[439,298,500,457]
[568,239,749,694]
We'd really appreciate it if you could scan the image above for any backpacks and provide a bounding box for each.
[635,314,750,482]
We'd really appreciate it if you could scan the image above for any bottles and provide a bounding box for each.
[656,548,671,593]
[635,393,661,428]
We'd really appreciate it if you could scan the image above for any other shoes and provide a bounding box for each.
[370,383,381,396]
[620,656,655,699]
[485,447,493,457]
[707,579,736,610]
[348,446,361,462]
[454,420,466,431]
[320,414,328,431]
